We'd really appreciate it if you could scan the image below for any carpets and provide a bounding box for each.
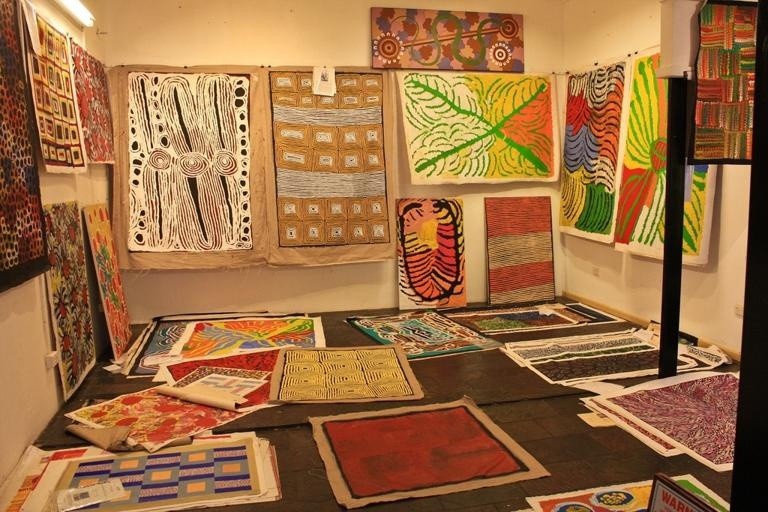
[30,298,738,448]
[178,392,733,512]
